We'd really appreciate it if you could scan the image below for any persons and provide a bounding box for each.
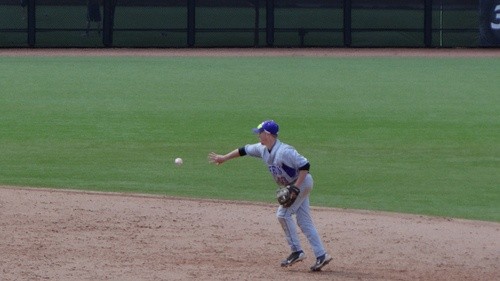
[208,120,333,271]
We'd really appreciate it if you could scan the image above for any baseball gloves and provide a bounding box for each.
[276,185,300,208]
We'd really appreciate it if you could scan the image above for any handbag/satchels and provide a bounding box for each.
[277,185,300,208]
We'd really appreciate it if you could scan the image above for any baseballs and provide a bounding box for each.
[175,158,182,166]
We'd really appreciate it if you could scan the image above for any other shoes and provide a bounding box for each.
[310,254,333,271]
[280,250,305,267]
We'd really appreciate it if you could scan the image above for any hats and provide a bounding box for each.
[253,120,279,133]
